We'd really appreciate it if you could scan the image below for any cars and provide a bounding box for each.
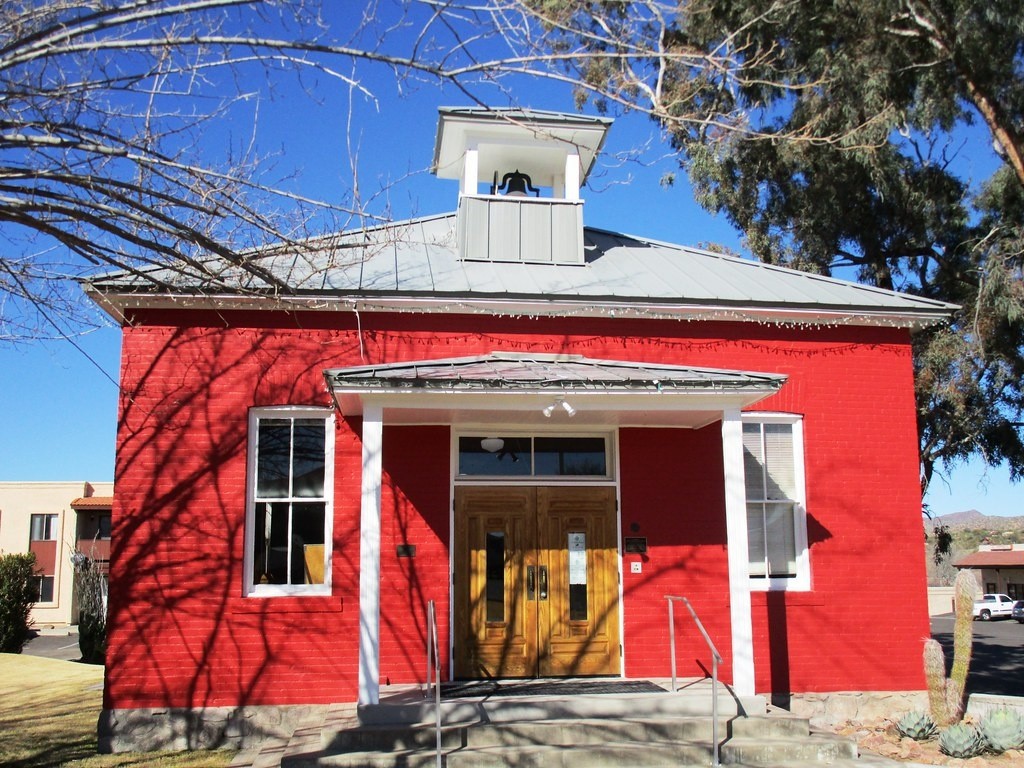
[1011,601,1024,623]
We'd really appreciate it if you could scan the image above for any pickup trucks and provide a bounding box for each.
[973,593,1019,621]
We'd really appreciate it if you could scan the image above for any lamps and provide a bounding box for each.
[542,396,577,417]
[480,436,505,453]
[496,448,521,464]
